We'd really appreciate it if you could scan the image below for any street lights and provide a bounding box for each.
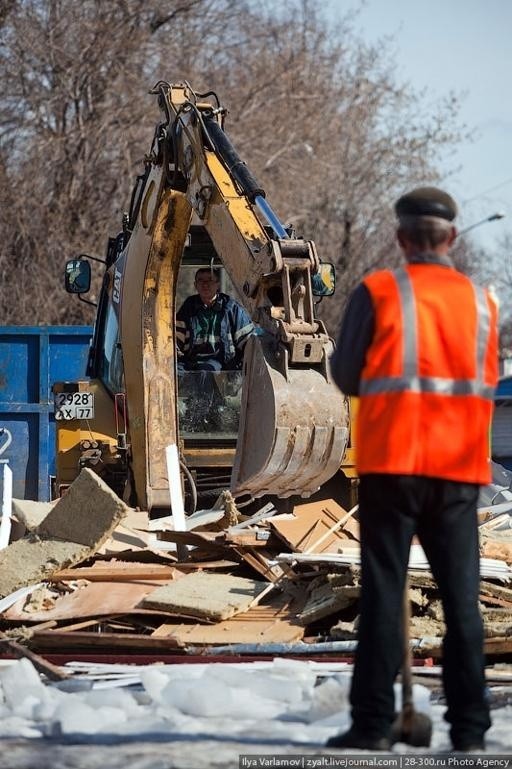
[456,209,503,237]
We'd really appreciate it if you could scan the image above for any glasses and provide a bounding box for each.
[196,279,217,285]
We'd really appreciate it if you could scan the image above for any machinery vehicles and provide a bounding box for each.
[50,79,362,520]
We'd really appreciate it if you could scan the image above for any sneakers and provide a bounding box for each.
[327,727,393,752]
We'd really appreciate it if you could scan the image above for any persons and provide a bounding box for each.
[330,187,499,751]
[177,267,258,408]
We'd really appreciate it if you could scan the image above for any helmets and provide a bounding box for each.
[395,187,456,221]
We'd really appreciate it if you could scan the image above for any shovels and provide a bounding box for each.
[386,569,433,751]
